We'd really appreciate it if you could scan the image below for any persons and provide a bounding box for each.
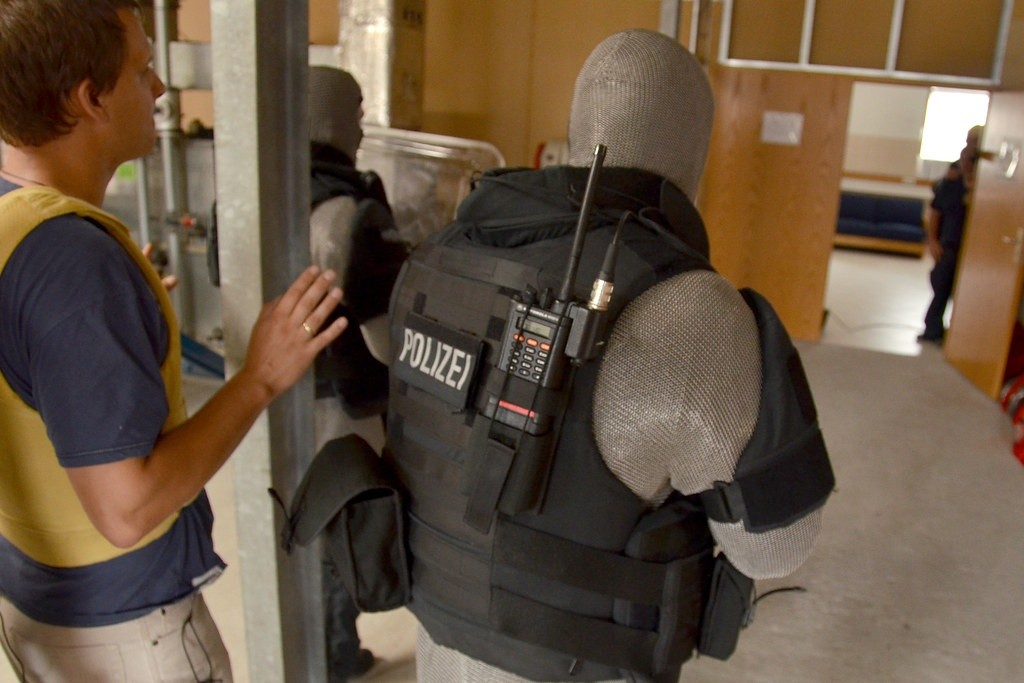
[296,64,412,679]
[353,26,837,683]
[1,0,348,682]
[916,125,985,344]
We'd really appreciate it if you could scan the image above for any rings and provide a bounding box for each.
[301,321,317,337]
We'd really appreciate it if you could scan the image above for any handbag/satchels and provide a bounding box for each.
[268,433,411,611]
